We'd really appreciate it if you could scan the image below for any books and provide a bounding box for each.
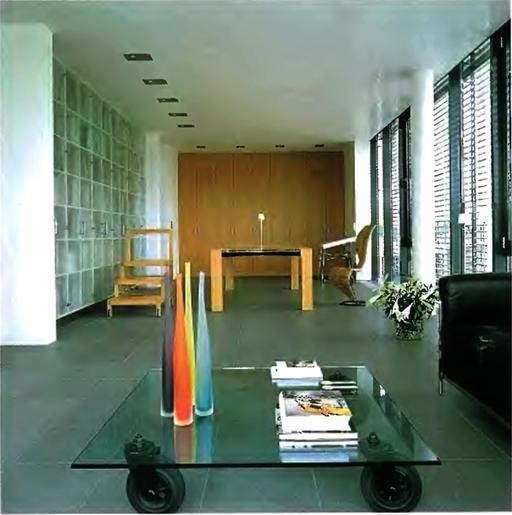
[270,359,359,451]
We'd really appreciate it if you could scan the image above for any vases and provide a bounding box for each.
[392,318,426,342]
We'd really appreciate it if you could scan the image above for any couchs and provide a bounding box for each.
[431,270,510,429]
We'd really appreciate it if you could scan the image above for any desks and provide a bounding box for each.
[318,234,357,284]
[209,248,315,314]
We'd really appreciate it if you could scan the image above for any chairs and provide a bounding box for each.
[324,222,379,309]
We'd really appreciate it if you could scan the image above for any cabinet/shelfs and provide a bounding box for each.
[53,54,147,326]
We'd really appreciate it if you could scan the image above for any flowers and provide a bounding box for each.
[366,271,441,327]
